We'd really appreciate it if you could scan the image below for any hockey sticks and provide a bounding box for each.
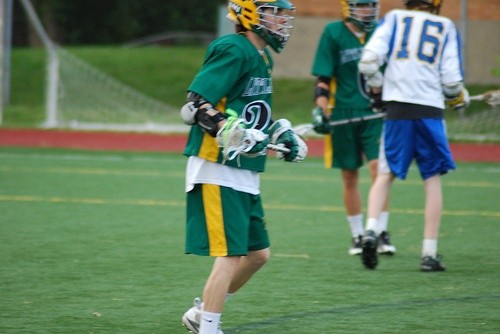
[217,109,291,166]
[329,90,500,125]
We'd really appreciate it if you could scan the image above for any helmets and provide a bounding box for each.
[403,0,442,11]
[226,0,296,54]
[339,0,380,32]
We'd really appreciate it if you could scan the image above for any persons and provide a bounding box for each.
[311,1,396,257]
[358,0,469,271]
[180,1,309,334]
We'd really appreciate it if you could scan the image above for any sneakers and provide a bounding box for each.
[181,298,224,334]
[376,231,396,255]
[361,230,379,269]
[420,254,446,271]
[349,234,363,255]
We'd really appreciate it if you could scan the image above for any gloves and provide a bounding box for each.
[449,88,470,111]
[311,106,331,134]
[216,122,269,158]
[268,118,308,162]
